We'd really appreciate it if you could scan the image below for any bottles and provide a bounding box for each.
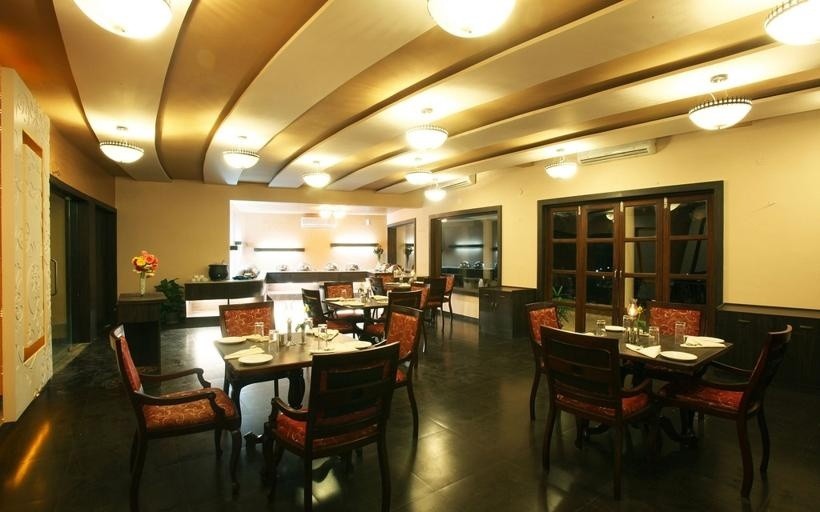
[360,293,366,303]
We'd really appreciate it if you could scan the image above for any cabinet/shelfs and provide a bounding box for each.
[711,303,820,389]
[479,286,536,340]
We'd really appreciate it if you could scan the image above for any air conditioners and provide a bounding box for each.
[619,165,621,166]
[579,142,657,165]
[301,217,336,230]
[441,175,475,189]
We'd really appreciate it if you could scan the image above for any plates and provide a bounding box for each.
[218,336,247,344]
[239,354,274,367]
[697,336,725,345]
[350,340,372,349]
[659,351,698,362]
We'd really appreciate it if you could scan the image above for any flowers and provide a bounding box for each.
[131,250,158,276]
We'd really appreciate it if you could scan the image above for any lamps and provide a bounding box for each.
[301,161,331,187]
[544,149,578,181]
[100,127,144,164]
[222,136,260,168]
[426,0,514,38]
[405,108,447,202]
[689,73,751,133]
[72,0,172,41]
[765,1,820,47]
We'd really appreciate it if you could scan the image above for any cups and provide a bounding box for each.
[254,321,266,341]
[267,329,279,355]
[318,323,329,350]
[387,290,392,301]
[594,314,686,349]
[339,288,348,303]
[189,271,208,282]
